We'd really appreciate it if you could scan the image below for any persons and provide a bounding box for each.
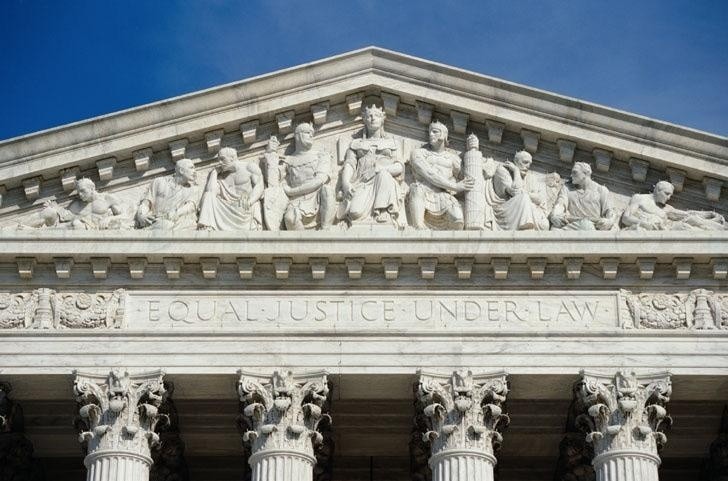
[43,177,124,231]
[197,147,264,231]
[621,180,702,230]
[342,104,407,231]
[136,158,200,230]
[408,121,475,231]
[265,122,337,231]
[493,151,550,231]
[550,161,615,231]
